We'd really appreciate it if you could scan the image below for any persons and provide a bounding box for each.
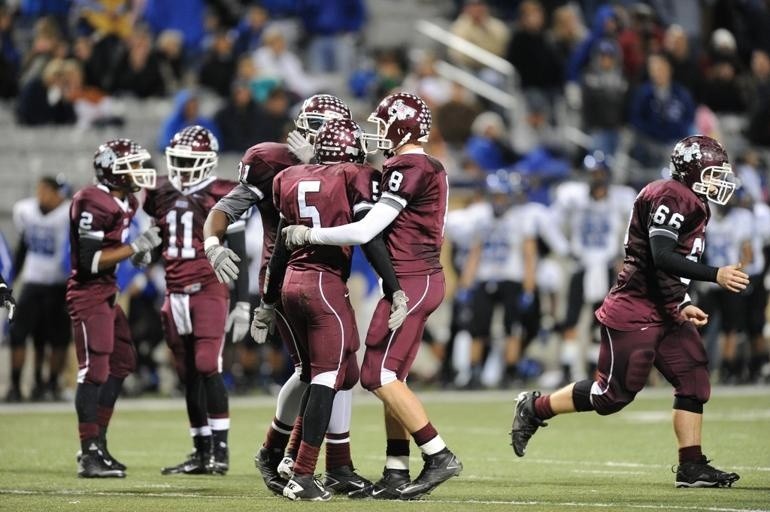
[130,125,252,479]
[1,275,17,324]
[65,137,162,480]
[509,133,750,488]
[535,152,638,390]
[696,182,766,388]
[275,90,463,502]
[200,94,373,502]
[454,169,538,390]
[0,172,76,404]
[351,37,467,143]
[253,118,409,500]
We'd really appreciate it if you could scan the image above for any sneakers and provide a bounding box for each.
[74,436,131,471]
[396,445,463,500]
[509,389,547,458]
[281,473,334,504]
[348,468,414,500]
[208,447,230,475]
[281,452,296,477]
[255,445,289,496]
[675,460,739,487]
[163,442,209,476]
[77,442,123,480]
[323,464,374,497]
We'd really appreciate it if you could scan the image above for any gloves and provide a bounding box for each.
[280,222,309,250]
[0,287,18,325]
[251,300,276,345]
[455,288,477,309]
[387,290,410,331]
[127,227,161,252]
[201,234,242,285]
[224,302,252,345]
[131,250,151,270]
[521,290,534,311]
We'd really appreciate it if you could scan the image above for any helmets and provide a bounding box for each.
[515,169,539,197]
[486,168,515,194]
[578,148,616,185]
[91,140,155,193]
[360,91,433,157]
[668,136,733,206]
[295,94,351,147]
[313,119,365,164]
[163,126,221,195]
